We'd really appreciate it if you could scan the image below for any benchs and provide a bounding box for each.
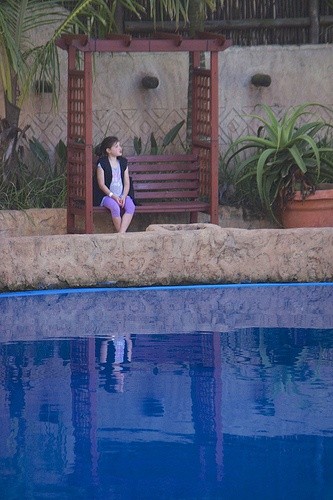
[70,152,210,234]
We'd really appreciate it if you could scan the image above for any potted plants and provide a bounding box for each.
[240,100,332,230]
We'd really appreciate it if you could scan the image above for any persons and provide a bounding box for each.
[93,136,135,232]
[98,334,136,393]
